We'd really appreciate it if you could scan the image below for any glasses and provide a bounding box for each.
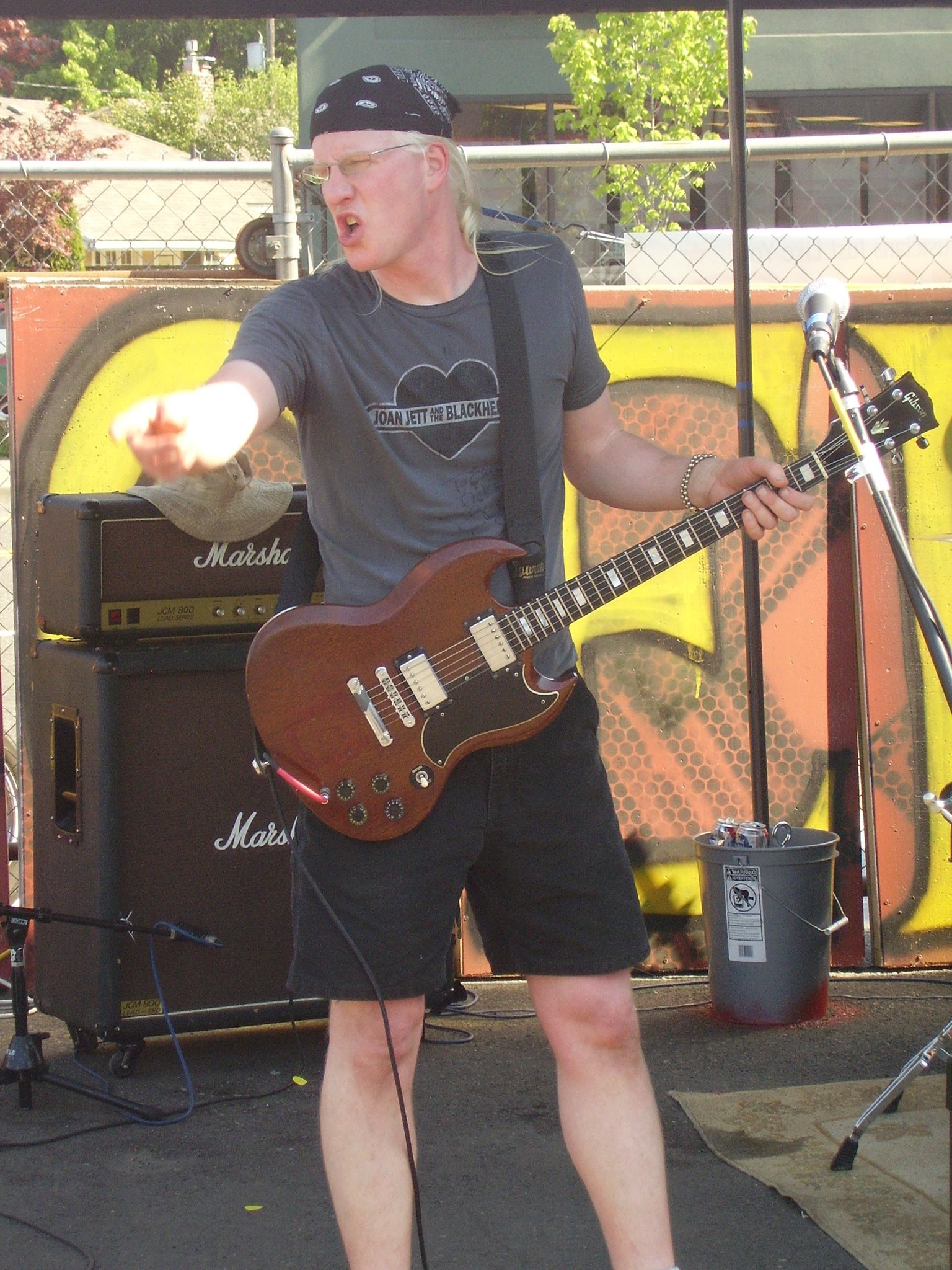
[296,142,429,202]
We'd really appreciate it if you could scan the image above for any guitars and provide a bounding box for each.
[242,370,943,845]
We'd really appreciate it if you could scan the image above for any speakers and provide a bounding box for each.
[18,634,455,1041]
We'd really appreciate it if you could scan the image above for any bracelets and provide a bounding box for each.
[680,454,717,512]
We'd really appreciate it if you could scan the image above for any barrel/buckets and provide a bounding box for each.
[691,826,838,1026]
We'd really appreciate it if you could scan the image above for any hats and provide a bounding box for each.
[120,449,295,542]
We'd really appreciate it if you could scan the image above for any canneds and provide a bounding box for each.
[709,818,770,849]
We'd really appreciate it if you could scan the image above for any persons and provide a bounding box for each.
[115,65,812,1270]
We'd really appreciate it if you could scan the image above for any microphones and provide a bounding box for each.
[797,276,850,359]
[170,920,223,949]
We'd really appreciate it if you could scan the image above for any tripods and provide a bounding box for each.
[0,900,203,1122]
